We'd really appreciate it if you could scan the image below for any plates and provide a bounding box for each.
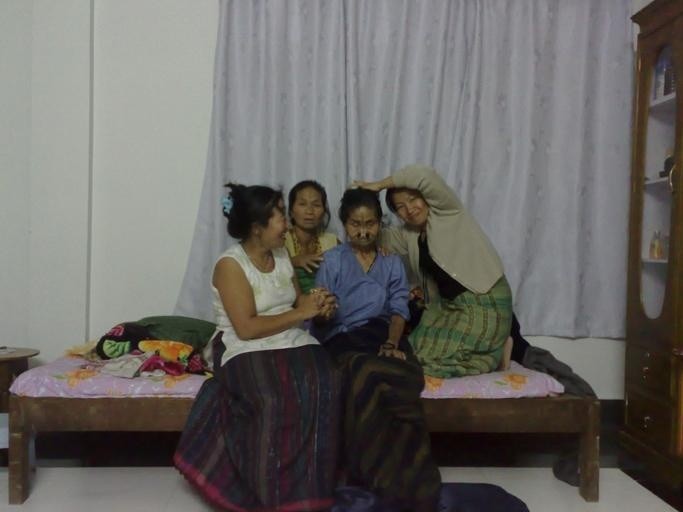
[619,0,683,512]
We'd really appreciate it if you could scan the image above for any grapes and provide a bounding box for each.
[0,347,41,472]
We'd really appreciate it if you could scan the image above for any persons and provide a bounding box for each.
[351,165,514,378]
[280,179,344,294]
[308,186,529,511]
[172,181,344,511]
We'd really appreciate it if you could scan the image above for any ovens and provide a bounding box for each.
[9,356,600,506]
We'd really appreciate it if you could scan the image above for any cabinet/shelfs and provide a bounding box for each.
[649,229,665,259]
[655,57,673,101]
